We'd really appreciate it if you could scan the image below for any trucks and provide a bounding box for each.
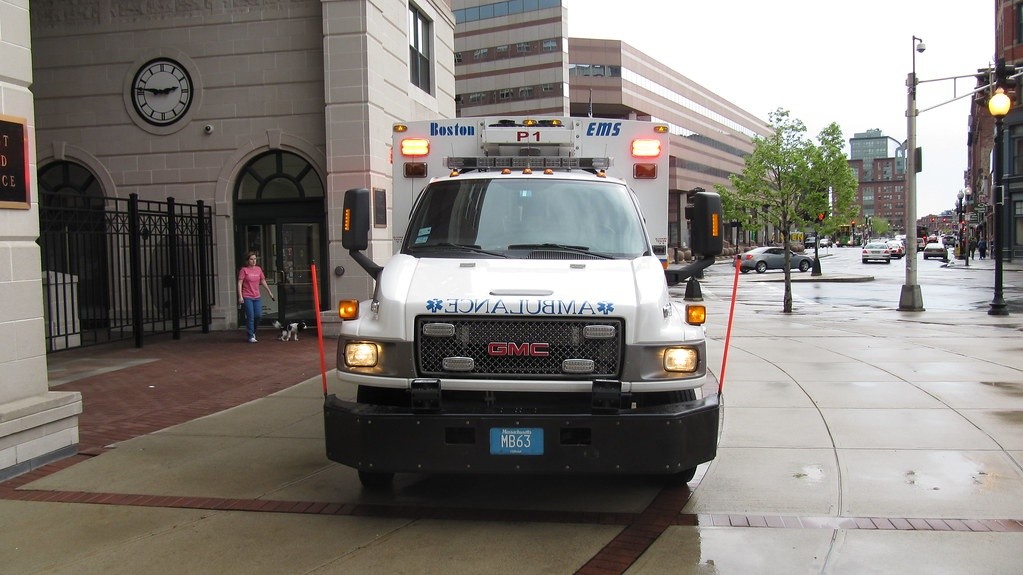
[316,115,721,494]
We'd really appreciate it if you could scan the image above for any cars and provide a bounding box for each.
[734,245,814,274]
[820,238,833,248]
[805,237,816,248]
[863,243,891,263]
[917,235,938,250]
[941,233,956,245]
[885,234,907,259]
[923,242,949,260]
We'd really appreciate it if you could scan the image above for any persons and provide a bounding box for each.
[237,252,274,342]
[978,237,987,260]
[970,237,978,260]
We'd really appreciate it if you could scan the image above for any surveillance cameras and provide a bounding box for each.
[204,125,213,133]
[916,44,926,53]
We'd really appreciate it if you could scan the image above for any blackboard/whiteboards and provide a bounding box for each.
[373,188,387,227]
[0,115,31,210]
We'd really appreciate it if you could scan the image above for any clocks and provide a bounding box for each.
[122,47,204,136]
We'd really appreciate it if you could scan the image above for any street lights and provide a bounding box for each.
[865,214,872,242]
[955,189,964,259]
[988,86,1010,316]
[964,184,972,266]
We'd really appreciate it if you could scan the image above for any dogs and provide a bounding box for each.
[272,320,308,341]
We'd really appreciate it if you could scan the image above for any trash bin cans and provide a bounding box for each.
[955,241,963,259]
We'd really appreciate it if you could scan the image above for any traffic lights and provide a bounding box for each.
[816,210,825,222]
[996,57,1016,93]
[975,70,989,106]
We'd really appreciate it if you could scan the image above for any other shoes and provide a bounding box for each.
[253,334,255,338]
[249,337,257,342]
